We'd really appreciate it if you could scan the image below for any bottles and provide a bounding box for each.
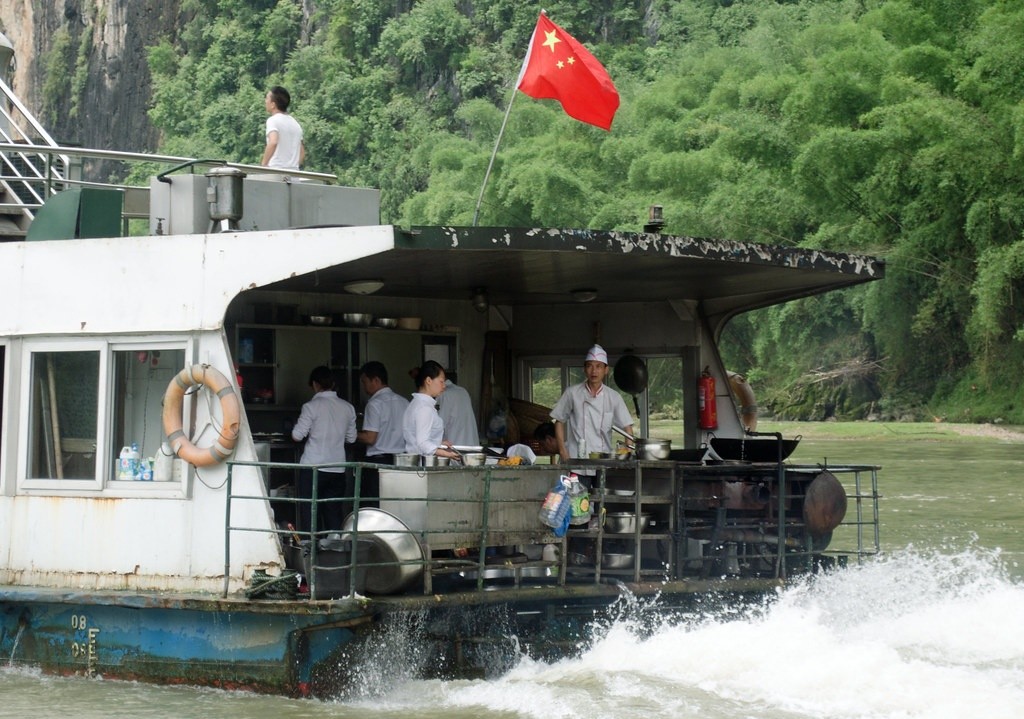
[537,473,591,529]
[152,442,173,481]
[543,543,561,577]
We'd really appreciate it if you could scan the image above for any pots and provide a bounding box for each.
[668,443,708,460]
[803,468,848,541]
[706,431,802,461]
[611,424,670,459]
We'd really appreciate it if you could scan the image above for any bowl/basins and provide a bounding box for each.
[339,507,428,595]
[466,566,549,593]
[591,552,634,569]
[394,452,488,467]
[604,511,657,533]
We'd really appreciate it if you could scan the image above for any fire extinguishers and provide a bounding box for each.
[699,364,718,430]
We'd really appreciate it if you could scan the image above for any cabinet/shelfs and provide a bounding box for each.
[232,323,462,413]
[569,457,674,583]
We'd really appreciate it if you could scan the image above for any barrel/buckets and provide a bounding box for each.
[299,539,373,599]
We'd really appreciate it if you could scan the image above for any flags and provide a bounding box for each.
[516,10,619,132]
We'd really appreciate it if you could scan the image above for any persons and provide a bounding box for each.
[403,360,460,460]
[291,364,357,540]
[357,361,409,507]
[262,86,305,171]
[533,420,560,455]
[435,380,479,447]
[549,344,634,492]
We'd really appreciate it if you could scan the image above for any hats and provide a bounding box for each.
[584,345,608,365]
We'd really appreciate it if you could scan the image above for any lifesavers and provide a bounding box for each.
[162,364,241,467]
[727,369,756,432]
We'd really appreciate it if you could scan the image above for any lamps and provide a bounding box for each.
[470,284,489,313]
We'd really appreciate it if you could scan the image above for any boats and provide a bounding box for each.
[0,76,886,702]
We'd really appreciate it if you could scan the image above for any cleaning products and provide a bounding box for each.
[116,442,155,481]
